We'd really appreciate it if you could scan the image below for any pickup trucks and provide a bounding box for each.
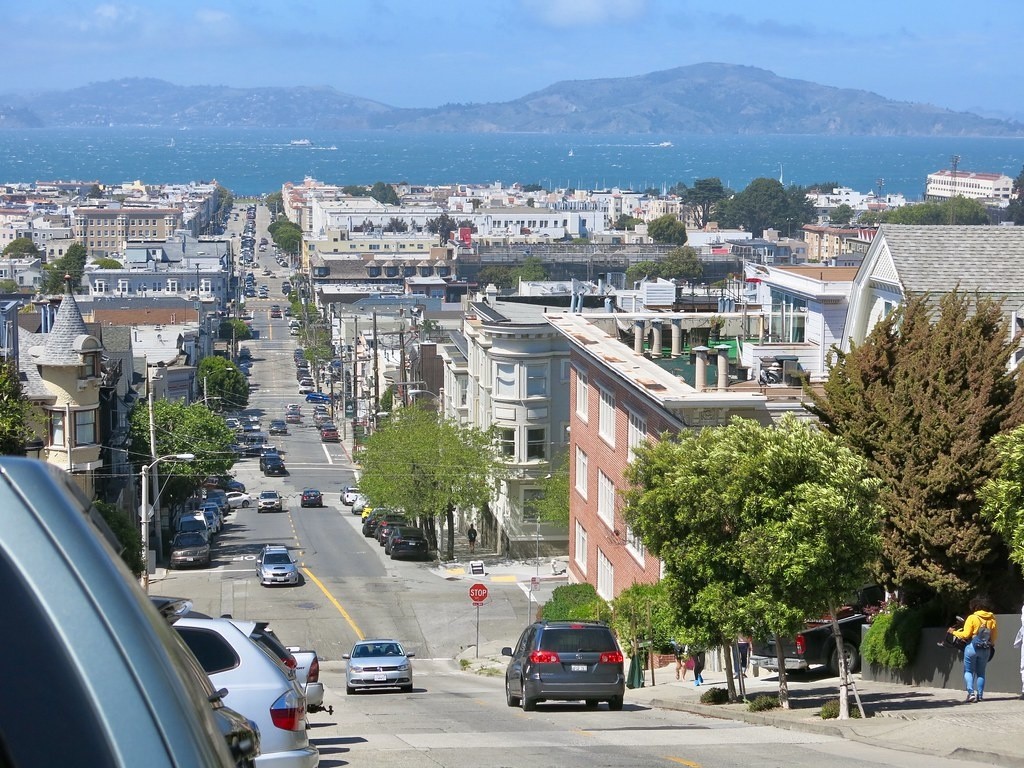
[285,646,334,714]
[750,584,886,677]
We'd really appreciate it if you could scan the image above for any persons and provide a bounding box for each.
[674,642,686,682]
[730,632,750,679]
[688,636,705,686]
[322,373,325,380]
[947,602,998,703]
[326,377,329,387]
[468,524,477,553]
[312,387,339,405]
[1014,605,1024,700]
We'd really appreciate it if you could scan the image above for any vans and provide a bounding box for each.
[0,455,236,768]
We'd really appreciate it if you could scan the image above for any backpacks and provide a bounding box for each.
[972,617,991,649]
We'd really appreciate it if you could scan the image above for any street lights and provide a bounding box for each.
[141,454,195,596]
[203,367,233,408]
[408,389,443,557]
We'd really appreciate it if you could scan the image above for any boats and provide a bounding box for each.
[292,139,311,145]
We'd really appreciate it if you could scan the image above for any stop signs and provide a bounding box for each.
[469,583,488,602]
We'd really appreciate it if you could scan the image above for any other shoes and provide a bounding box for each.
[978,699,982,701]
[965,693,975,702]
[698,674,703,683]
[695,680,699,686]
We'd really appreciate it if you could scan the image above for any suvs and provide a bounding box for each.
[177,511,213,545]
[362,508,407,539]
[174,617,325,768]
[260,454,285,476]
[229,621,324,729]
[169,530,211,569]
[502,618,625,711]
[256,490,282,513]
[352,494,373,515]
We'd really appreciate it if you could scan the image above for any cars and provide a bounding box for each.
[205,492,230,515]
[285,404,301,422]
[258,284,268,298]
[385,526,428,559]
[236,346,253,376]
[170,626,262,768]
[312,404,339,442]
[241,315,253,337]
[251,262,258,267]
[269,209,274,222]
[203,477,246,492]
[271,304,282,318]
[377,522,407,546]
[259,246,265,251]
[294,347,316,394]
[361,501,374,522]
[340,485,363,506]
[282,281,290,295]
[224,492,251,509]
[269,418,288,435]
[271,240,289,267]
[224,415,278,459]
[245,271,256,297]
[306,391,331,404]
[289,319,306,336]
[343,638,415,695]
[260,238,267,244]
[300,489,323,507]
[239,204,256,265]
[262,270,269,276]
[198,502,223,525]
[269,273,275,278]
[204,512,222,534]
[255,544,298,586]
